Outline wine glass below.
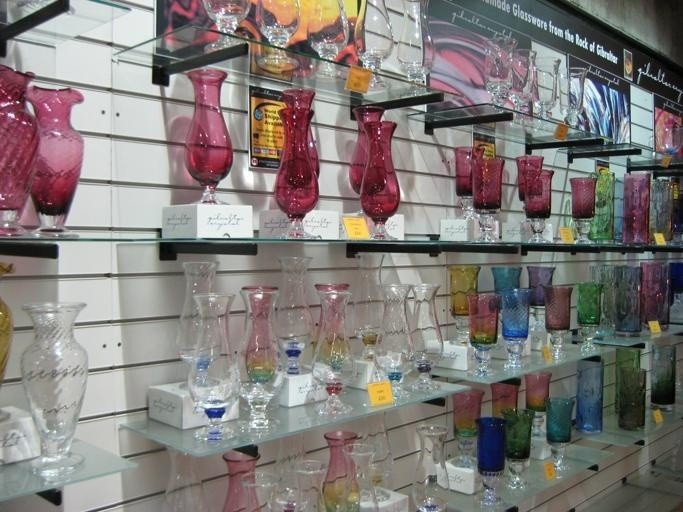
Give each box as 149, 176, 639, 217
186, 72, 234, 208
548, 398, 576, 477
491, 378, 521, 422
239, 286, 282, 437
447, 141, 599, 248
540, 284, 572, 356
350, 106, 385, 217
373, 284, 414, 402
311, 278, 354, 418
469, 294, 498, 385
0, 61, 39, 238
576, 360, 602, 439
273, 107, 326, 243
257, 4, 301, 73
189, 294, 239, 444
405, 285, 439, 395
503, 407, 535, 493
499, 287, 529, 369
576, 283, 602, 365
352, 248, 388, 369
283, 89, 321, 212
306, 1, 351, 81
359, 122, 401, 242
272, 253, 314, 373
355, 3, 393, 91
524, 372, 553, 416
22, 86, 87, 240
201, 1, 253, 53
490, 266, 524, 324
179, 261, 220, 390
19, 301, 91, 477
472, 416, 507, 510
445, 264, 480, 344
0, 1, 603, 512
149, 425, 455, 512
450, 390, 481, 470
397, 2, 438, 98
524, 266, 555, 333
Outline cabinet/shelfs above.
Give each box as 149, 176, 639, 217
0, 1, 683, 512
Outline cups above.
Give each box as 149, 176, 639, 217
592, 175, 675, 435
